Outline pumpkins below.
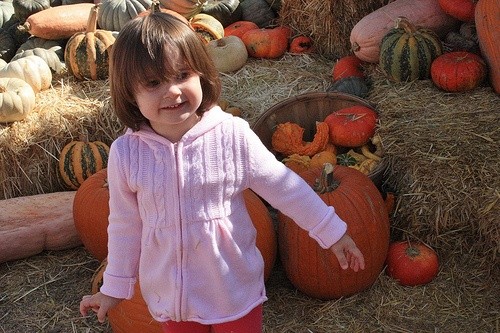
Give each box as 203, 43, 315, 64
0, 105, 438, 333
0, 0, 316, 125
327, 0, 500, 102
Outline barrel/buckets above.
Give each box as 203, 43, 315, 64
251, 92, 389, 190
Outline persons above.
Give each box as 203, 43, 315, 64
79, 13, 366, 333
